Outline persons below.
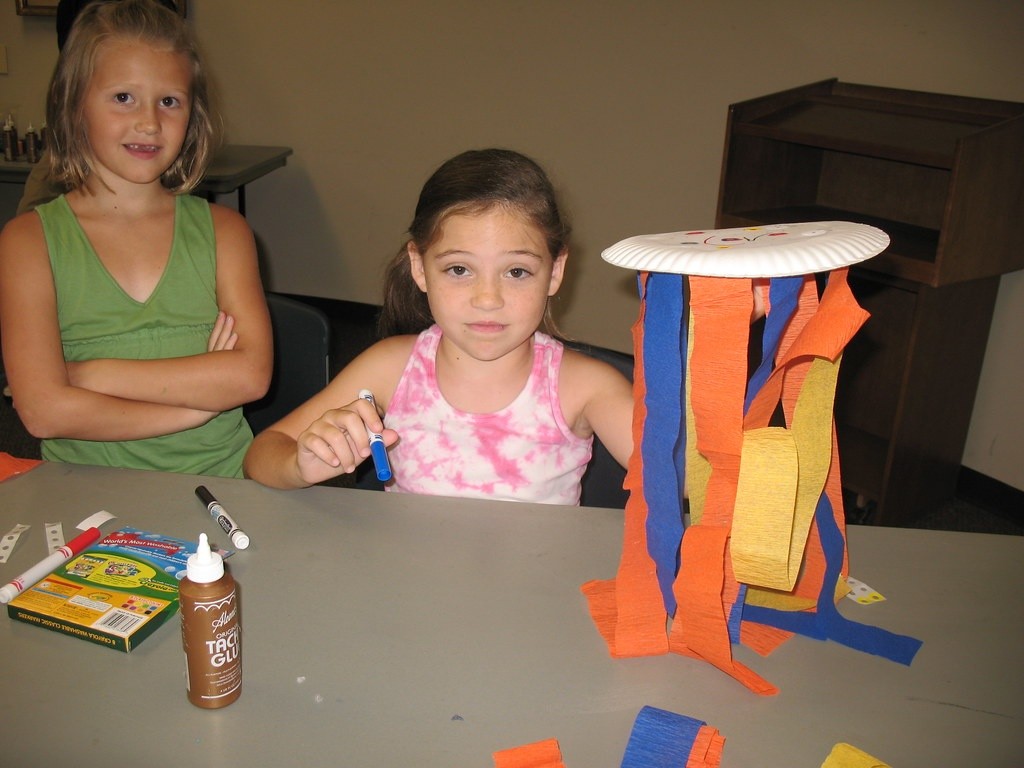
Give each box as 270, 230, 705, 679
17, 0, 189, 220
0, 0, 274, 480
241, 144, 767, 509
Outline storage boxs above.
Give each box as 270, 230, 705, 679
7, 524, 236, 655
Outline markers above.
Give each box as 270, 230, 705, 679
0, 527, 101, 604
194, 485, 250, 550
358, 388, 393, 482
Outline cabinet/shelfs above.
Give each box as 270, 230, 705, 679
714, 77, 1024, 525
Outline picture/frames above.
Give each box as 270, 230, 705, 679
15, 0, 187, 19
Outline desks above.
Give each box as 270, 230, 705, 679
0, 452, 1024, 768
0, 144, 294, 216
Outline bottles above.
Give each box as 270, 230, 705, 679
179, 534, 242, 709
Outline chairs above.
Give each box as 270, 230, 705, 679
16, 293, 343, 489
355, 335, 635, 509
